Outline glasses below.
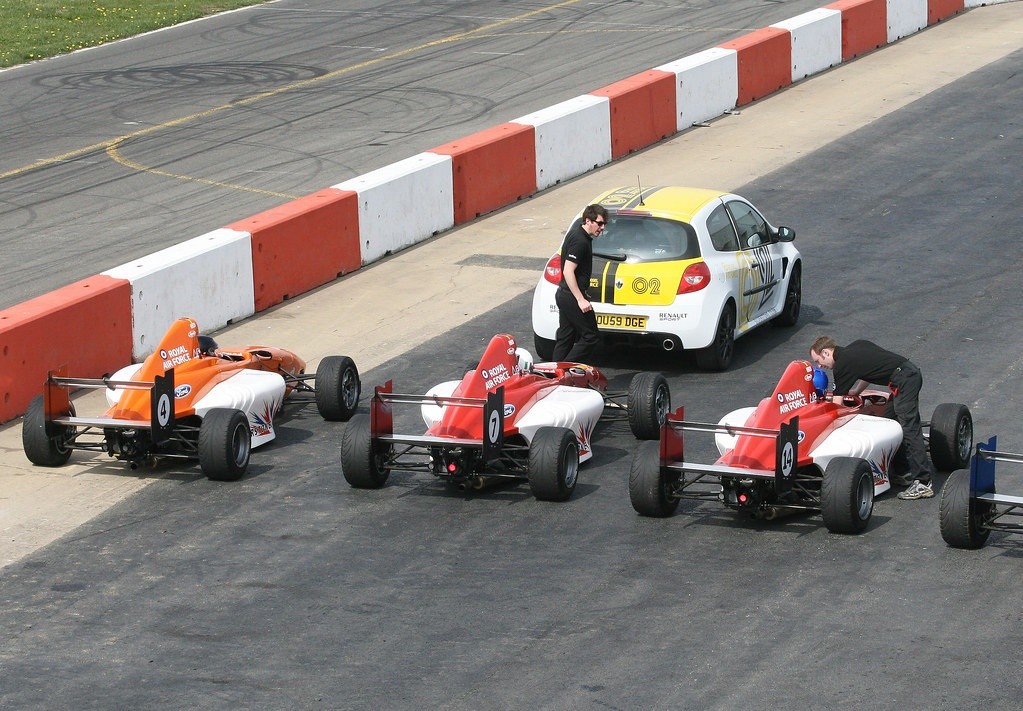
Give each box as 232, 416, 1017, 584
594, 220, 607, 226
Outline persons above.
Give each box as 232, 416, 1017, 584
514, 348, 533, 371
810, 337, 934, 500
198, 336, 218, 357
553, 204, 608, 363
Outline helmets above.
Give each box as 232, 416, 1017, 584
197, 336, 219, 357
515, 347, 534, 372
813, 368, 829, 396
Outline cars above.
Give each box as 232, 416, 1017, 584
531, 186, 802, 372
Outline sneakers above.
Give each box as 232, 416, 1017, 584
896, 479, 934, 499
889, 470, 912, 486
